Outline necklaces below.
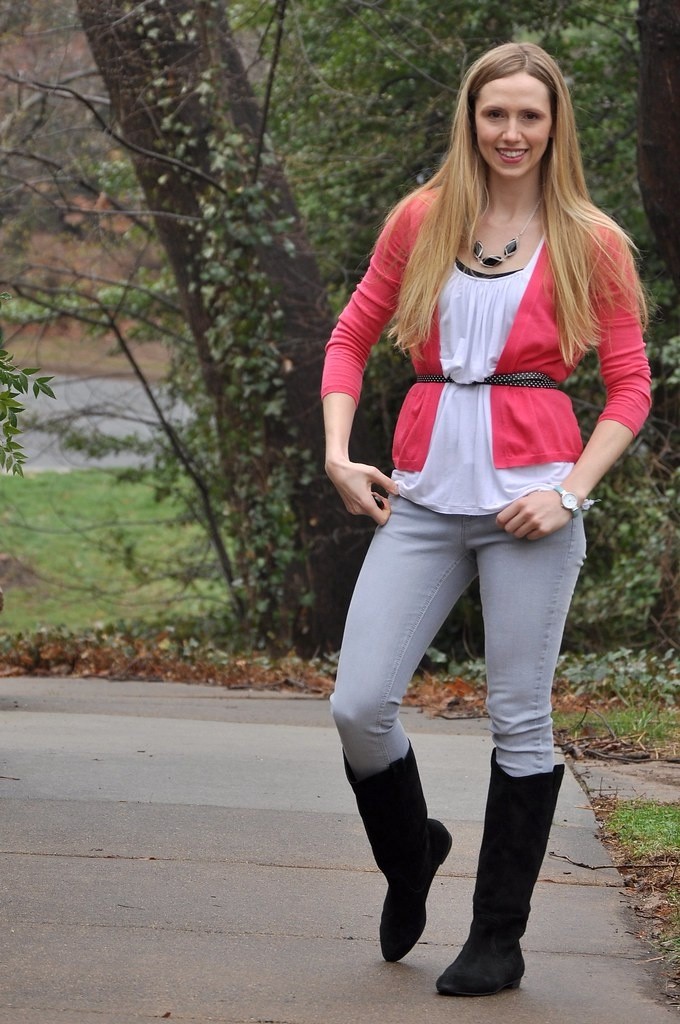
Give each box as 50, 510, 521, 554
472, 195, 540, 268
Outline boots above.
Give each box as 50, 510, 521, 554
436, 747, 565, 996
342, 737, 452, 963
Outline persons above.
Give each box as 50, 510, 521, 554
320, 42, 653, 994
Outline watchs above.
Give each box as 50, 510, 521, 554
553, 485, 581, 518
